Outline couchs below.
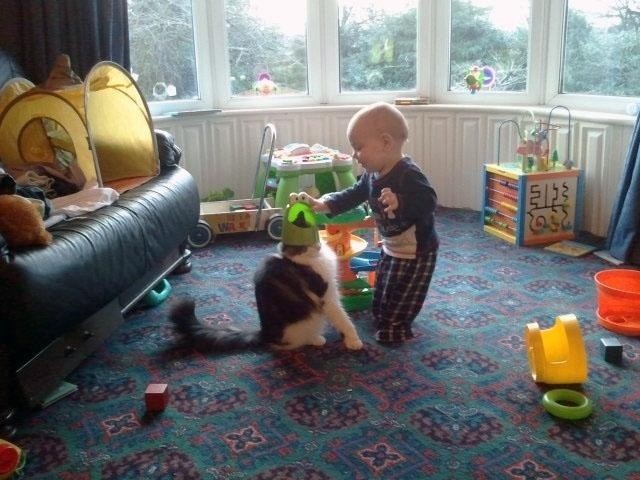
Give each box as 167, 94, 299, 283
1, 47, 201, 415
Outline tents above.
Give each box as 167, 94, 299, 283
0, 61, 162, 191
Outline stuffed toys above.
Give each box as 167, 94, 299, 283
466, 65, 494, 95
0, 195, 52, 251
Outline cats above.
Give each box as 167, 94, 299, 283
164, 211, 364, 356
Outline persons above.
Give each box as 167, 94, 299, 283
309, 102, 440, 344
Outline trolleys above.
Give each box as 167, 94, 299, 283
186, 124, 282, 246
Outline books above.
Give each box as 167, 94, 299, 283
544, 240, 598, 257
